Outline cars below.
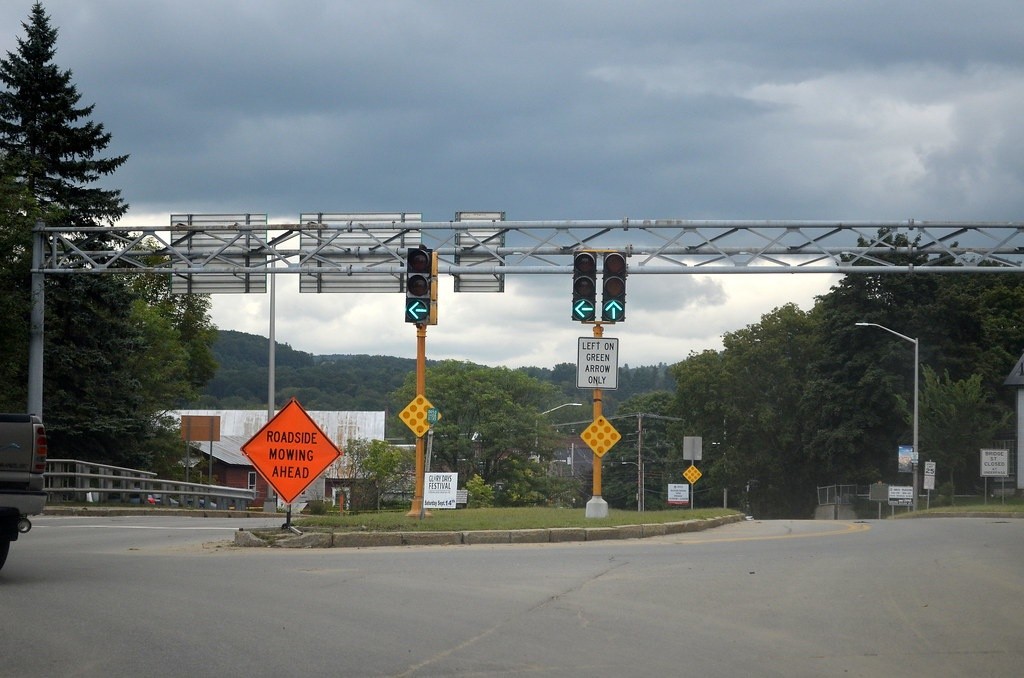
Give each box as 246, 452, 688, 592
58, 491, 217, 510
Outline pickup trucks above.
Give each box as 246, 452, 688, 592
0, 412, 49, 570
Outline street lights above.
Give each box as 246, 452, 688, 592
535, 402, 583, 446
572, 249, 627, 324
855, 322, 920, 513
404, 246, 433, 324
